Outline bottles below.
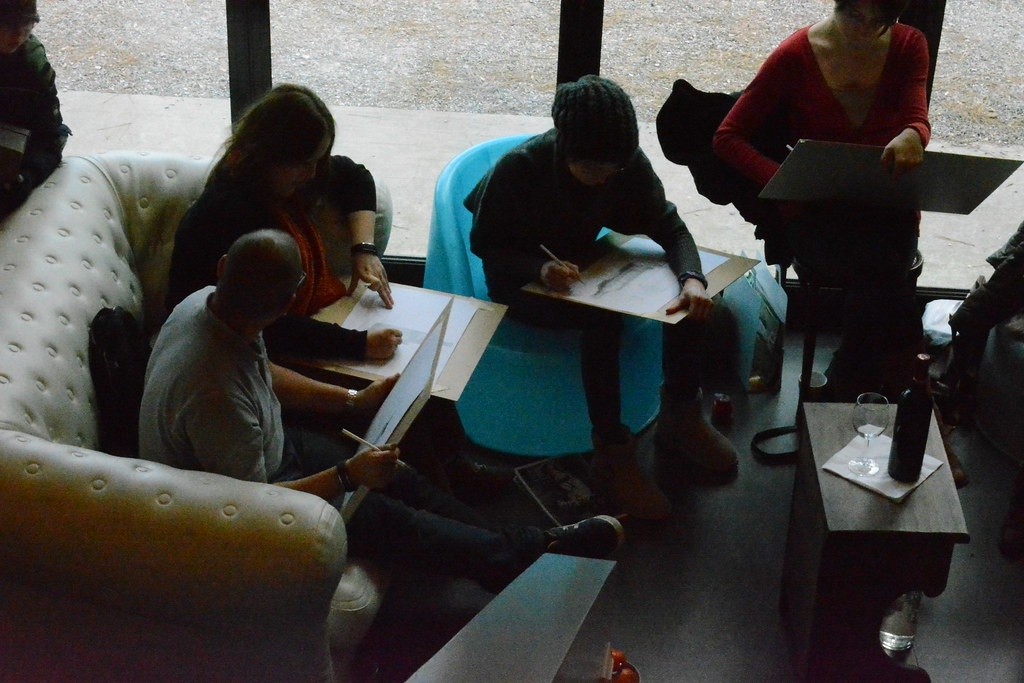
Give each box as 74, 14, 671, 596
888, 352, 934, 483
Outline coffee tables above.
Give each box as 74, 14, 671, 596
784, 396, 971, 681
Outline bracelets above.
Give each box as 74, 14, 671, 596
350, 242, 378, 255
677, 270, 708, 285
337, 460, 355, 494
343, 389, 358, 420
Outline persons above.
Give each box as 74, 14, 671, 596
463, 74, 742, 519
1, 0, 72, 228
138, 230, 626, 599
714, 0, 972, 490
165, 82, 405, 367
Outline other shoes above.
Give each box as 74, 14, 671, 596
654, 382, 740, 474
589, 426, 667, 525
828, 339, 966, 487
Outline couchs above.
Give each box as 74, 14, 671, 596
0, 151, 394, 683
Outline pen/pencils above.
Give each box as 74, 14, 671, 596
341, 428, 412, 471
539, 243, 585, 284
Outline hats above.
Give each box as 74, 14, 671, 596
553, 74, 641, 166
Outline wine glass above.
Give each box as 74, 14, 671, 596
848, 392, 891, 476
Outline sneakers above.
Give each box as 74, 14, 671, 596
546, 514, 625, 561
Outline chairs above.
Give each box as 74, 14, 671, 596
766, 243, 926, 422
420, 130, 664, 458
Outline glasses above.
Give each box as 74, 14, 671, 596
297, 270, 306, 288
